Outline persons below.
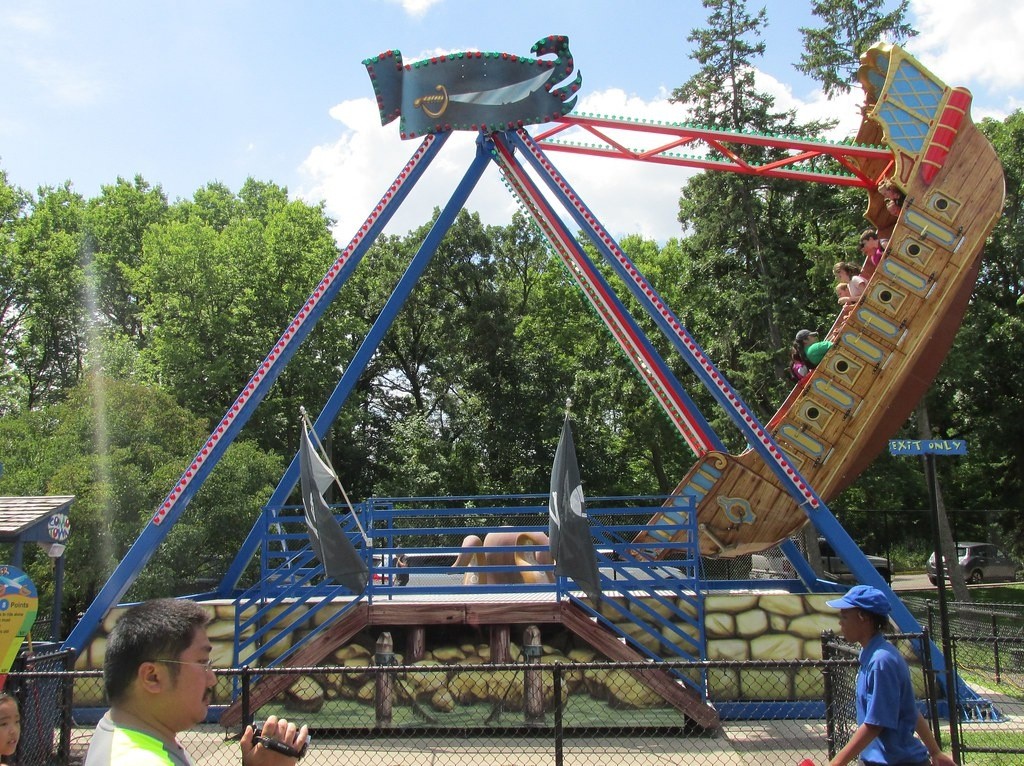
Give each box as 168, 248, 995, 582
785, 176, 906, 379
84, 596, 308, 766
392, 544, 410, 586
0, 693, 22, 766
823, 584, 958, 766
299, 419, 371, 597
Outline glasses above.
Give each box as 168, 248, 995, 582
149, 659, 213, 673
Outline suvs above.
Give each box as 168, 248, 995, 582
752, 536, 893, 586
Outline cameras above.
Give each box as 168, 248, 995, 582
251, 721, 312, 759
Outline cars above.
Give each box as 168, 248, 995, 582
926, 542, 1024, 586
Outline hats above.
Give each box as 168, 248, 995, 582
825, 584, 891, 616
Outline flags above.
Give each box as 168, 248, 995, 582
548, 413, 602, 606
